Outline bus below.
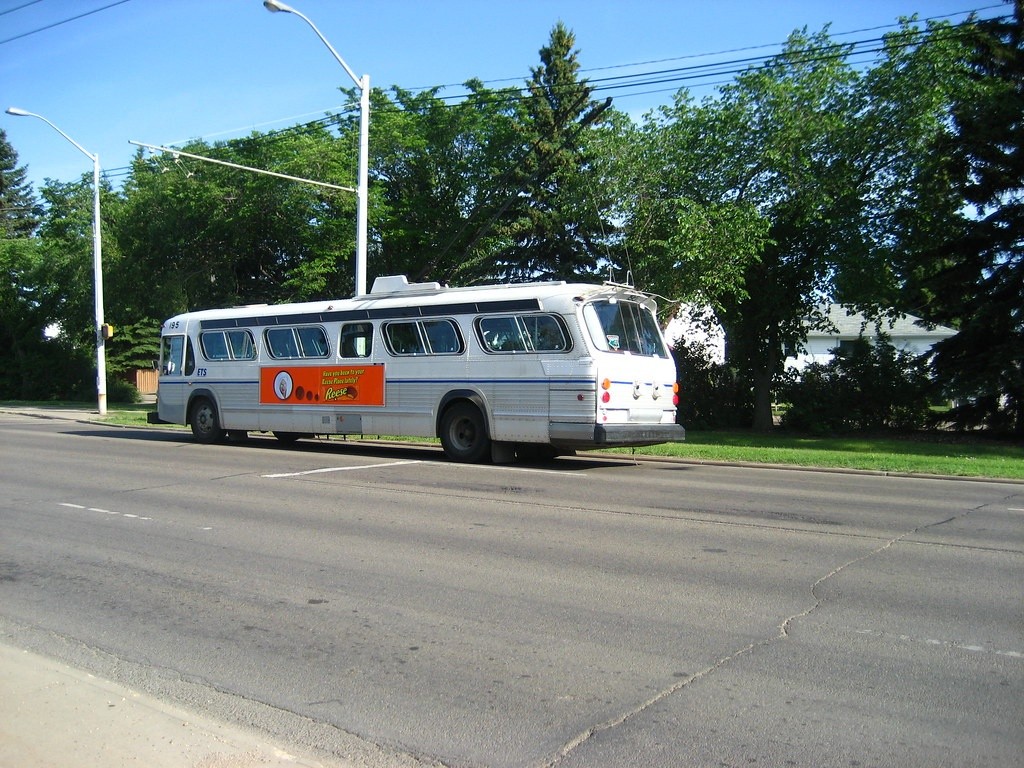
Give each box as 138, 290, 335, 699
145, 273, 687, 465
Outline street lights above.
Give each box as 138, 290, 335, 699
261, 0, 371, 358
5, 107, 108, 418
